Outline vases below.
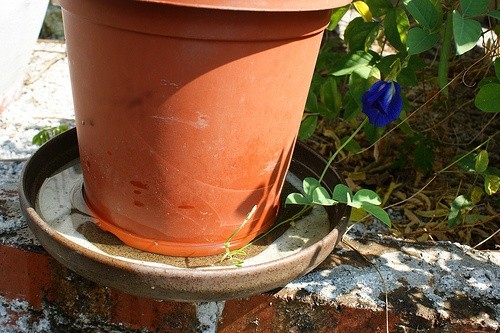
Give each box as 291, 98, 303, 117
58, 0, 354, 257
